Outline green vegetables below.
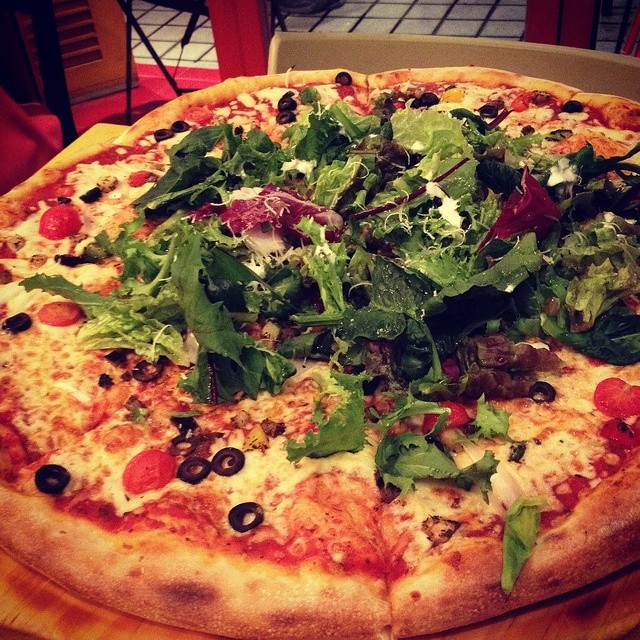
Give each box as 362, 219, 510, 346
17, 86, 640, 596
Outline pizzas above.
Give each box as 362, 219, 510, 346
0, 65, 640, 639
